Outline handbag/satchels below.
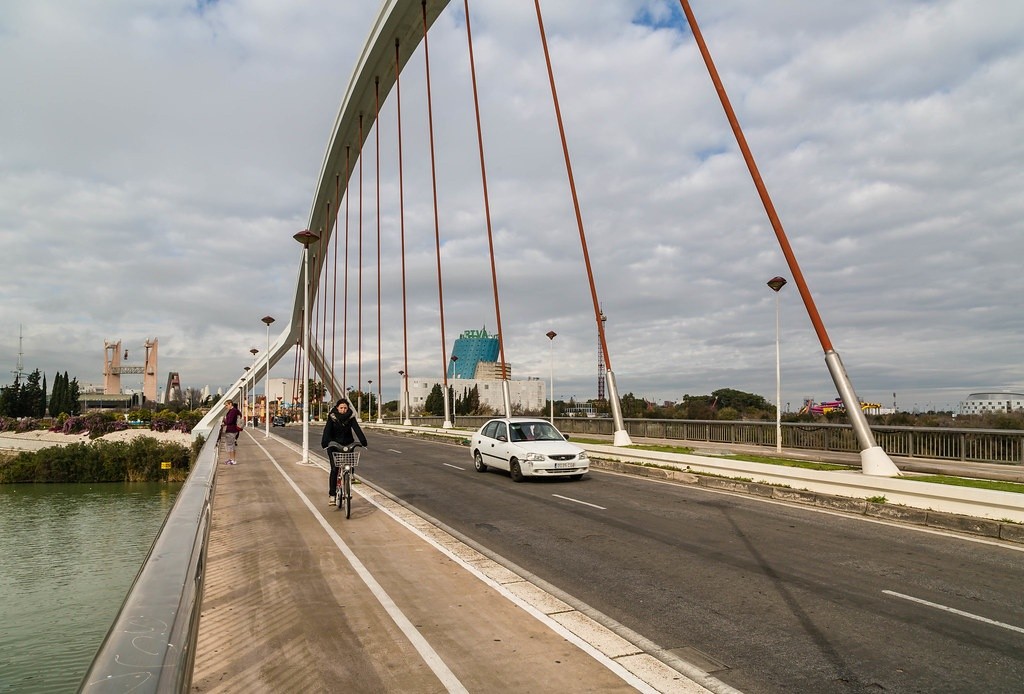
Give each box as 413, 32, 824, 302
236, 414, 244, 432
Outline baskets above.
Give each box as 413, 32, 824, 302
332, 452, 360, 467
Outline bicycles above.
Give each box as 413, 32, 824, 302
323, 442, 368, 519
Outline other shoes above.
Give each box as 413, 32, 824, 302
328, 495, 336, 506
351, 478, 361, 484
224, 460, 236, 465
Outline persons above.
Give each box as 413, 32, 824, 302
321, 399, 366, 506
222, 399, 242, 464
530, 424, 543, 439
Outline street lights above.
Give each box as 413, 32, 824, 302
244, 367, 251, 426
368, 379, 373, 422
398, 370, 404, 424
250, 348, 259, 427
546, 330, 557, 426
260, 315, 275, 438
239, 386, 242, 412
451, 355, 458, 415
766, 276, 788, 453
241, 378, 246, 422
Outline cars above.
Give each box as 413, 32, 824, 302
272, 417, 285, 427
470, 418, 590, 484
